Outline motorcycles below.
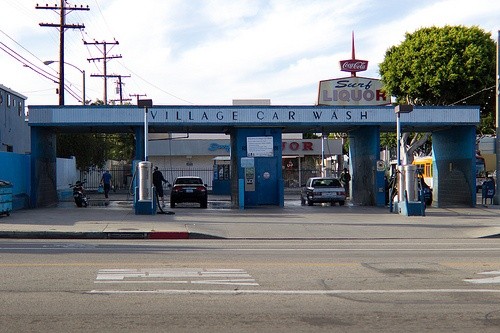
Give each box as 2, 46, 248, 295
69, 178, 88, 208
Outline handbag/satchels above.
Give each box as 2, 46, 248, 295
98, 184, 104, 193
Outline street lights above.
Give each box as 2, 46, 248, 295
43, 60, 86, 105
395, 103, 414, 165
137, 99, 153, 160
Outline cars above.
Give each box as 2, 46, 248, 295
168, 176, 208, 209
417, 177, 433, 206
301, 176, 346, 206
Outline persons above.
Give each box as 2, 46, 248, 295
384, 176, 390, 206
340, 168, 350, 197
100, 170, 114, 198
153, 167, 168, 207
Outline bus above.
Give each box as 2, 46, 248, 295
390, 150, 487, 193
